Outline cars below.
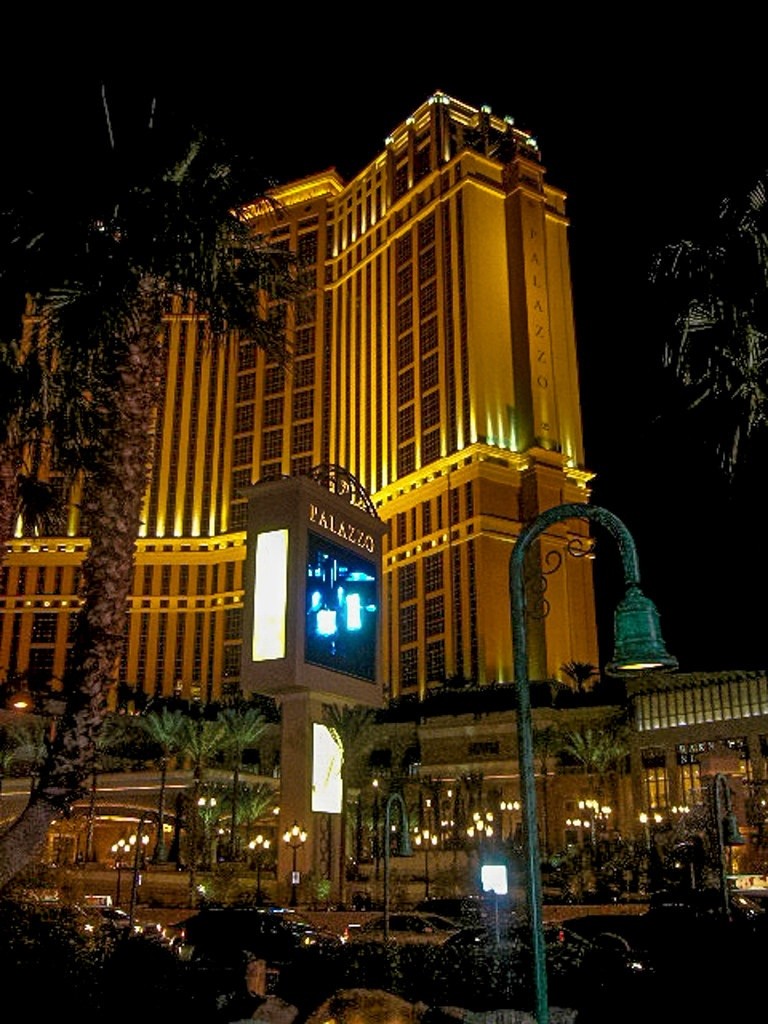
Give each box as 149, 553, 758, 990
166, 907, 359, 987
429, 919, 658, 1007
1, 889, 229, 1023
343, 909, 459, 943
411, 897, 489, 925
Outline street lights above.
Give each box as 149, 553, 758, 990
468, 809, 496, 896
247, 835, 273, 908
508, 500, 681, 1024
381, 793, 417, 942
110, 838, 131, 906
710, 771, 747, 930
283, 820, 311, 908
414, 824, 439, 901
128, 806, 170, 935
